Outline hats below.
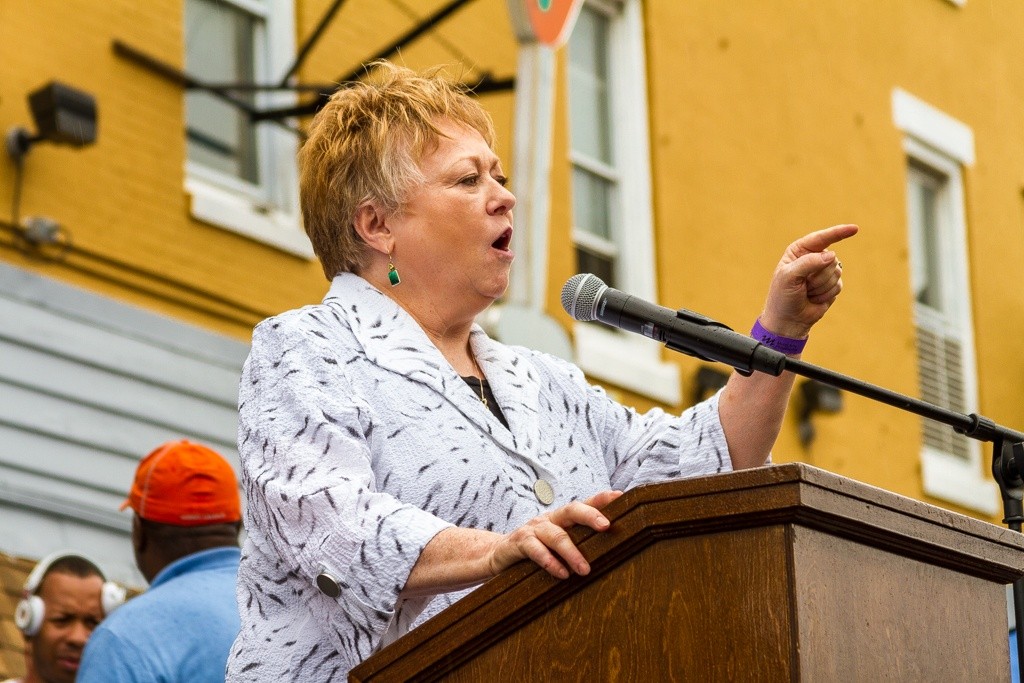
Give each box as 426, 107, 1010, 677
120, 439, 242, 525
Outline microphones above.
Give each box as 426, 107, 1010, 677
560, 273, 787, 378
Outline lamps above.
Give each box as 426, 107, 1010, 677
797, 380, 842, 446
7, 83, 96, 158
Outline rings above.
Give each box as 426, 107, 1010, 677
838, 260, 844, 269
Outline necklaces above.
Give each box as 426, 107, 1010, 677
453, 352, 488, 408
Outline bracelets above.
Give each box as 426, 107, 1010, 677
750, 317, 809, 356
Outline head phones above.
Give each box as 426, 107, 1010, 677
14, 552, 126, 637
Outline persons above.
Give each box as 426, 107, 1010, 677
0, 555, 127, 683
221, 60, 858, 683
76, 441, 249, 683
